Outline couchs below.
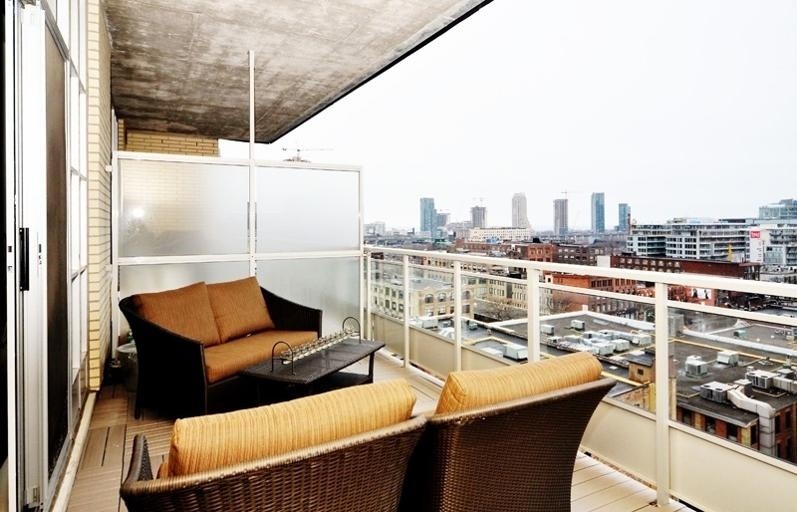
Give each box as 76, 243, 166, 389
118, 277, 322, 420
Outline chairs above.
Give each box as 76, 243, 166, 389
399, 377, 620, 512
120, 414, 428, 512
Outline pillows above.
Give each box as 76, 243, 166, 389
435, 353, 602, 419
169, 380, 417, 472
132, 276, 273, 349
